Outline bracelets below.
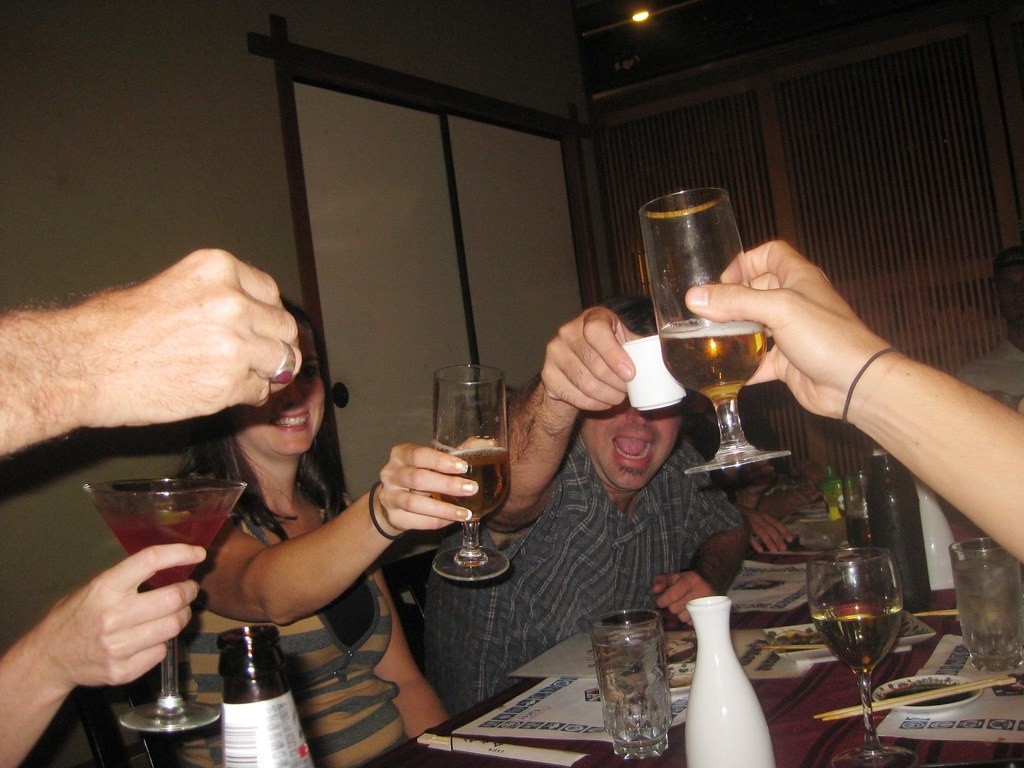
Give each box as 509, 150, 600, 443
842, 348, 899, 424
368, 481, 404, 539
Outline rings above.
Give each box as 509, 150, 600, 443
268, 338, 296, 384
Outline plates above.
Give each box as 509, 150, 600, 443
872, 675, 982, 719
763, 611, 936, 661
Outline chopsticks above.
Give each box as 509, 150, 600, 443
755, 644, 831, 649
813, 676, 1016, 722
912, 609, 958, 616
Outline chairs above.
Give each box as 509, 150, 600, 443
69, 548, 439, 768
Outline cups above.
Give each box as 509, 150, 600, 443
622, 334, 687, 411
949, 537, 1024, 675
590, 608, 673, 760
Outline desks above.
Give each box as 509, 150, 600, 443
349, 512, 1024, 768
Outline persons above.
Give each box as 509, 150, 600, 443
174, 294, 479, 767
686, 237, 1023, 566
415, 295, 817, 715
1, 247, 306, 453
957, 245, 1024, 398
0, 543, 206, 768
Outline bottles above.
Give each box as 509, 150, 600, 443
913, 476, 958, 590
841, 469, 876, 551
818, 466, 847, 521
216, 624, 318, 768
864, 453, 931, 614
685, 595, 777, 767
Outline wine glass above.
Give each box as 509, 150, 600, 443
638, 187, 791, 475
82, 478, 249, 732
431, 364, 511, 582
808, 547, 918, 768
